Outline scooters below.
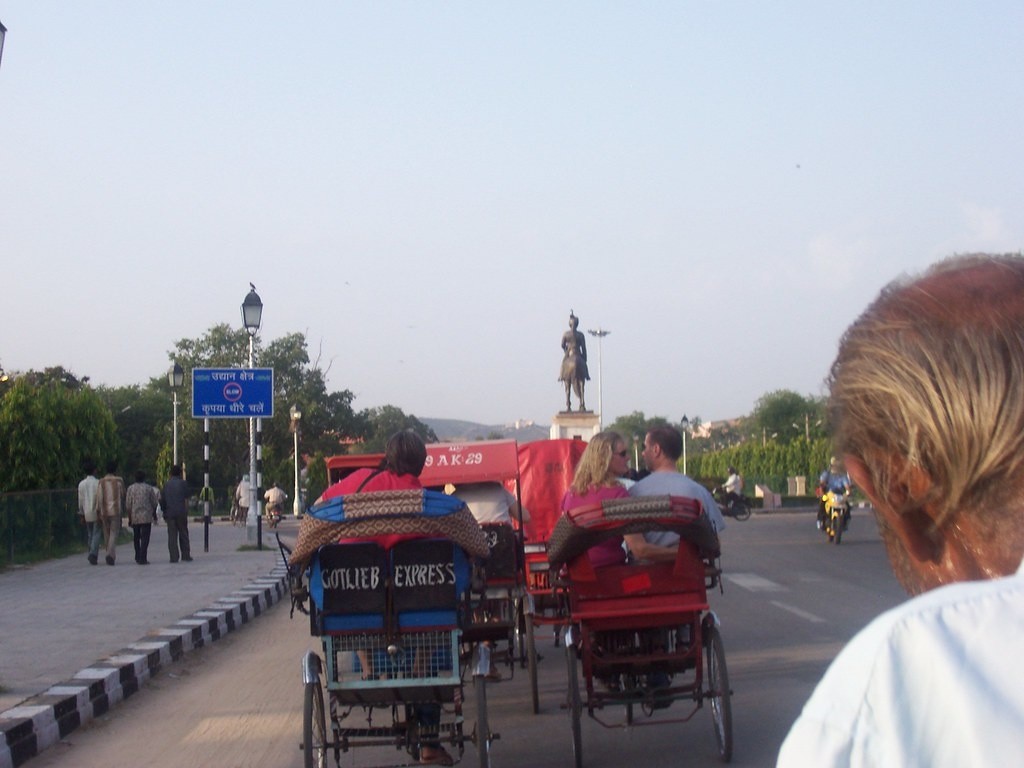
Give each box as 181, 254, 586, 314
268, 492, 288, 529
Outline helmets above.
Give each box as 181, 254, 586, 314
274, 482, 279, 487
830, 456, 837, 467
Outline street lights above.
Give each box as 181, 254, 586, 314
792, 413, 823, 441
681, 414, 690, 475
166, 362, 185, 466
632, 431, 641, 473
240, 282, 264, 550
587, 328, 611, 434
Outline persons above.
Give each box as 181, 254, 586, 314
150, 479, 162, 515
816, 456, 851, 528
125, 470, 158, 565
718, 466, 743, 508
628, 426, 725, 685
560, 432, 676, 567
451, 482, 530, 531
236, 475, 250, 508
162, 465, 193, 563
264, 482, 288, 519
78, 464, 100, 564
776, 252, 1024, 768
311, 432, 456, 768
95, 462, 126, 566
558, 315, 590, 412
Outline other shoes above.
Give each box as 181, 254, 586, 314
418, 745, 454, 765
817, 520, 824, 530
361, 674, 377, 681
648, 671, 672, 710
182, 554, 193, 561
105, 555, 114, 565
136, 558, 147, 564
88, 555, 97, 565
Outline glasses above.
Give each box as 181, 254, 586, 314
614, 449, 626, 458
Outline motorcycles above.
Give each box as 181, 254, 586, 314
817, 487, 854, 543
710, 486, 751, 521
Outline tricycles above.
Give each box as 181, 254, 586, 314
292, 439, 737, 768
